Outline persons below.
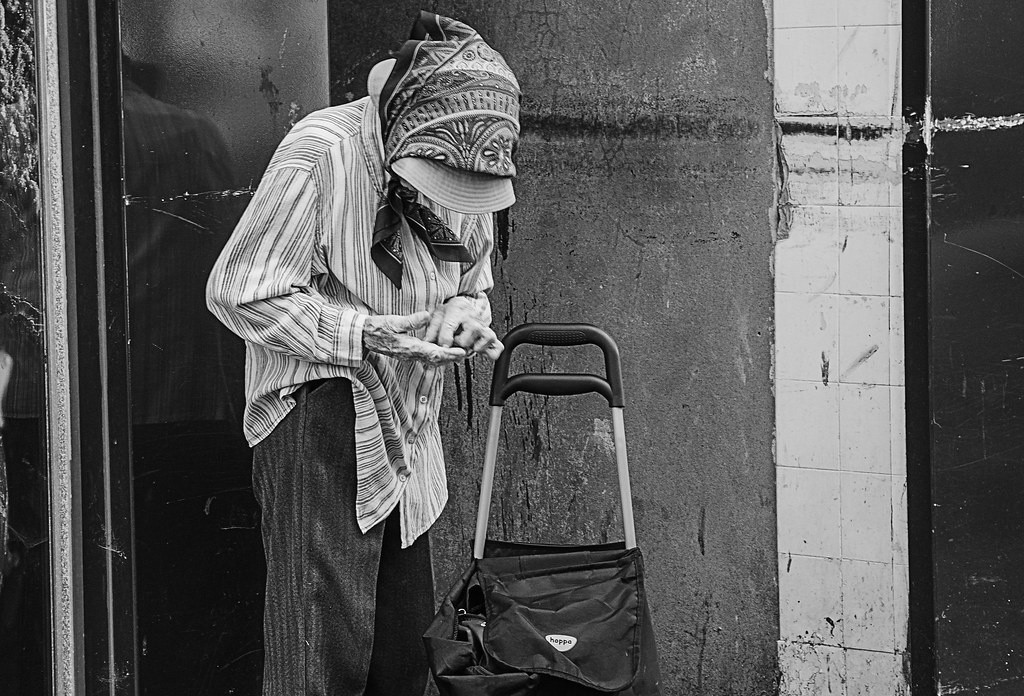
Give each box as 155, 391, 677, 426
204, 9, 525, 695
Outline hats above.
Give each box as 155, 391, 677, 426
368, 59, 517, 213
